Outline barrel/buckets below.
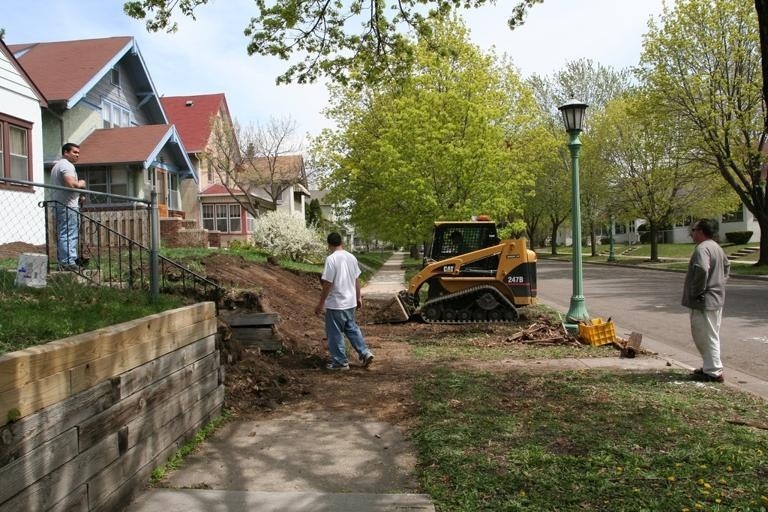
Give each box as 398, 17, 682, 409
14, 253, 49, 290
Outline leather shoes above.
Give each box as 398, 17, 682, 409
693, 368, 724, 383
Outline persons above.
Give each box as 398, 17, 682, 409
315, 233, 374, 370
680, 218, 731, 384
441, 230, 471, 268
48, 143, 88, 271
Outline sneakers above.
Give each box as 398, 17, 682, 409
56, 264, 84, 271
361, 352, 374, 368
326, 362, 350, 371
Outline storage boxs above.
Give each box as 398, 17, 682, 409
579, 318, 617, 348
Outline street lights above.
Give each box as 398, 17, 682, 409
606, 199, 617, 261
557, 90, 591, 335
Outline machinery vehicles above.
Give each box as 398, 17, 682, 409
367, 220, 537, 325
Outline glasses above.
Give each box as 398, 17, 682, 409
691, 228, 702, 231
